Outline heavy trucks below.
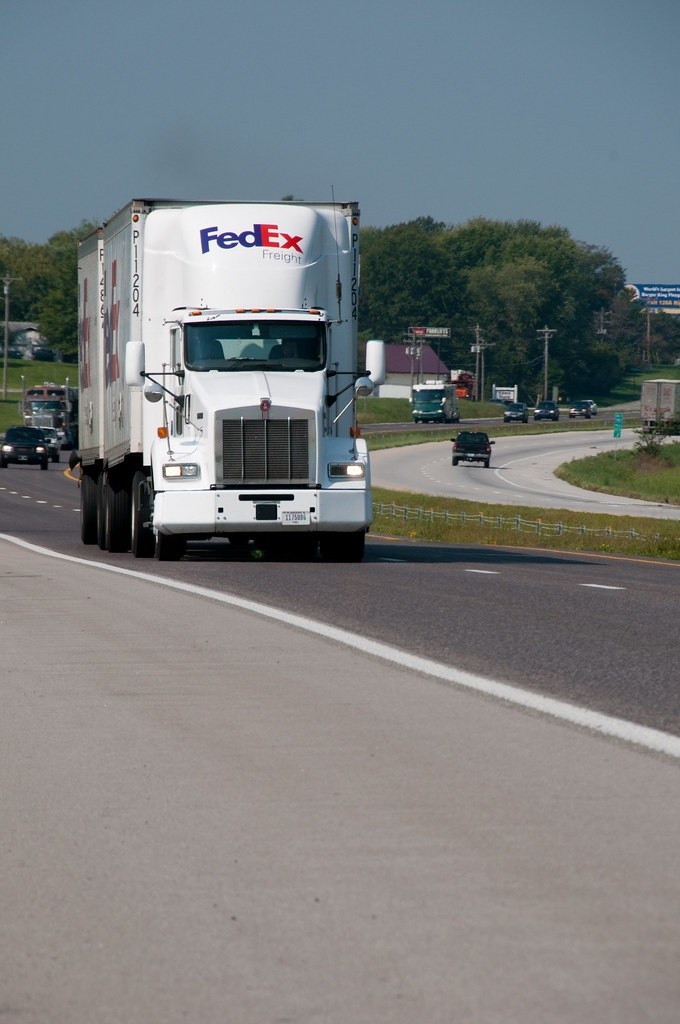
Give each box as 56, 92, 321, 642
73, 197, 390, 561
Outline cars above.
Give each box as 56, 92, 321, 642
449, 430, 496, 470
569, 400, 592, 420
582, 400, 599, 415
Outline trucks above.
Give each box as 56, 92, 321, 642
411, 379, 461, 424
19, 382, 78, 451
640, 379, 680, 434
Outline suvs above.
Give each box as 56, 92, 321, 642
534, 400, 562, 422
0, 427, 51, 471
504, 402, 530, 423
26, 425, 62, 463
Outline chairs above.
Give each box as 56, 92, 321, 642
268, 345, 301, 359
193, 341, 224, 360
